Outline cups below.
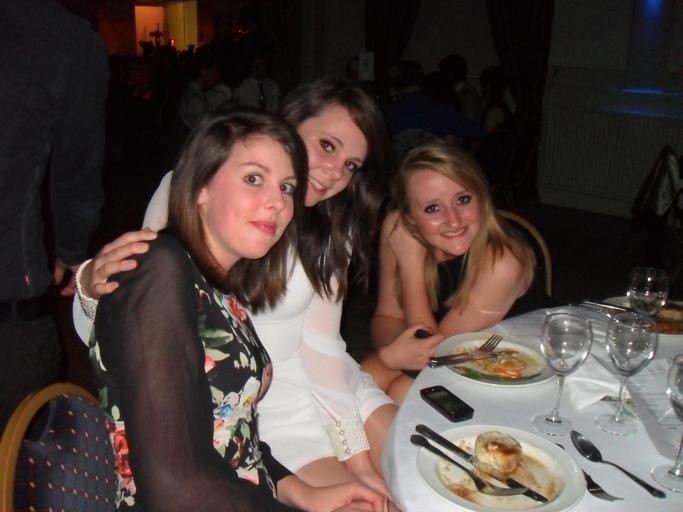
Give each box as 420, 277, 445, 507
628, 266, 669, 318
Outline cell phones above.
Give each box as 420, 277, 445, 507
420, 384, 475, 422
400, 329, 433, 378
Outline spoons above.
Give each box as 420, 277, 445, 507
570, 429, 666, 499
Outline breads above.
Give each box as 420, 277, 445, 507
475, 431, 522, 474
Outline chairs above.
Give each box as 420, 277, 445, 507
480, 110, 541, 206
1, 382, 122, 512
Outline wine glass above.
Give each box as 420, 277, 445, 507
597, 311, 660, 436
652, 351, 683, 493
532, 312, 594, 437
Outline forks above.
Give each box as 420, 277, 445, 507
429, 333, 503, 363
403, 432, 528, 498
553, 441, 625, 502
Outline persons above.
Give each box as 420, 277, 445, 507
345, 45, 518, 206
366, 142, 536, 407
2, 3, 112, 509
71, 78, 397, 512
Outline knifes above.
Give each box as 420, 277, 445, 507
415, 423, 549, 504
426, 349, 520, 369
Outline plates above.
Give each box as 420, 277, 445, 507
600, 295, 683, 338
436, 331, 558, 387
401, 424, 587, 512
